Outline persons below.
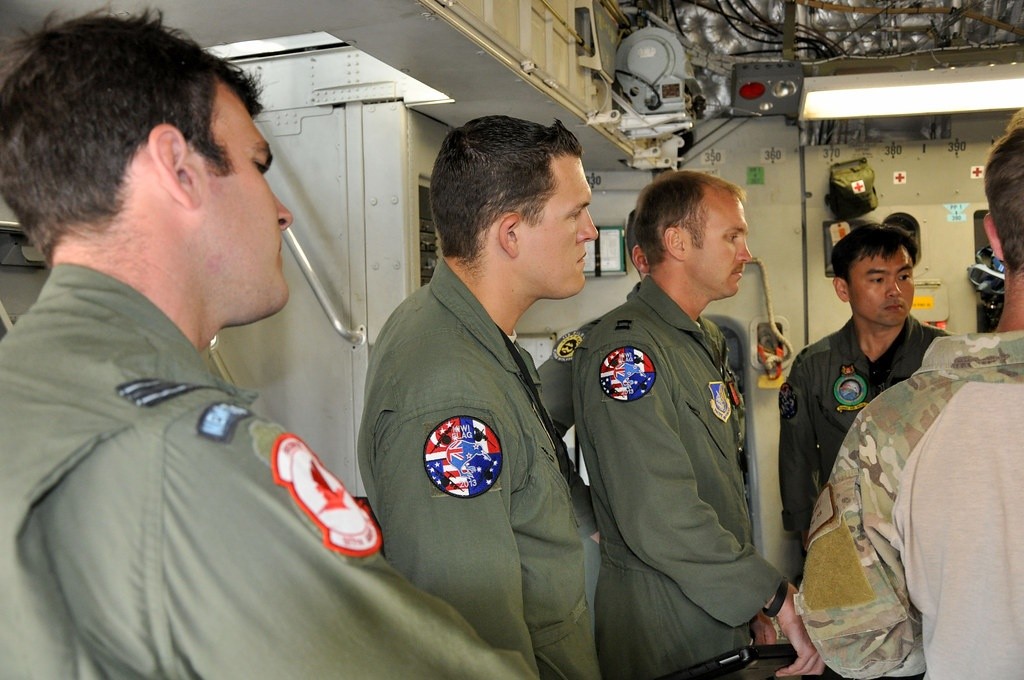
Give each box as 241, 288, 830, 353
537, 210, 651, 542
1, 13, 540, 680
358, 116, 601, 680
794, 105, 1024, 680
572, 171, 823, 680
778, 224, 953, 551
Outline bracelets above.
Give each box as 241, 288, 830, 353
762, 575, 788, 617
